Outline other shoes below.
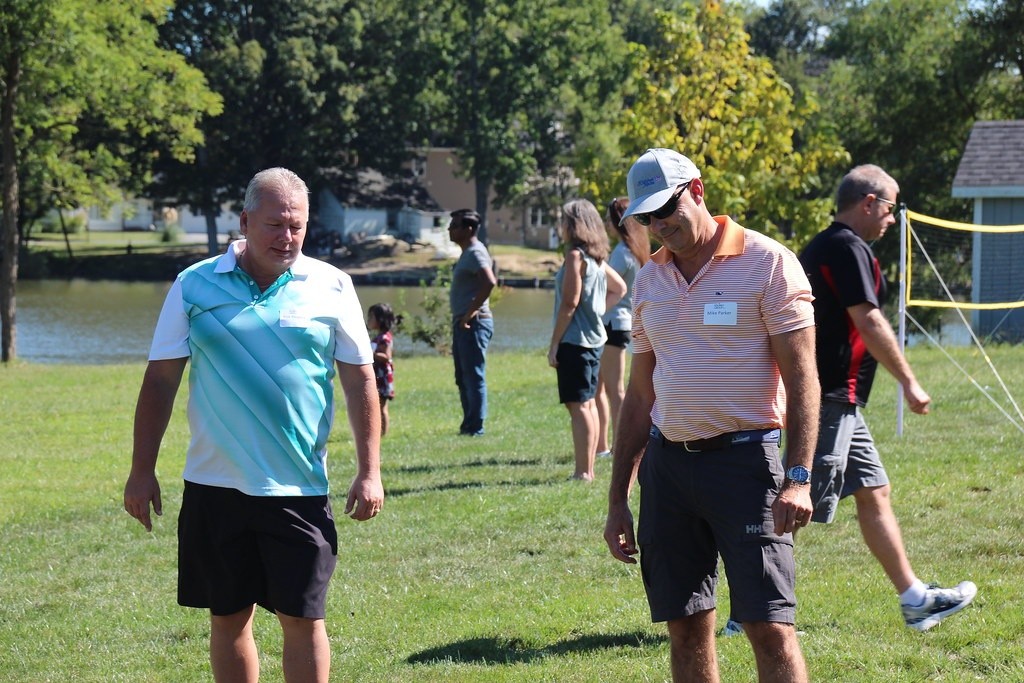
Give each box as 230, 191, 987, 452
596, 449, 611, 458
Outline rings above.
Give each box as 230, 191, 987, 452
796, 520, 802, 523
374, 509, 381, 512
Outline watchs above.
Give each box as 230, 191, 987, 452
786, 465, 811, 485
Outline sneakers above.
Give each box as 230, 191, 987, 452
903, 580, 978, 631
724, 621, 741, 636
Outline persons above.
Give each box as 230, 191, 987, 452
124, 166, 384, 683
604, 148, 822, 683
721, 165, 978, 636
366, 303, 402, 435
547, 199, 650, 481
447, 209, 497, 435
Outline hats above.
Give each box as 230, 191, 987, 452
619, 148, 702, 226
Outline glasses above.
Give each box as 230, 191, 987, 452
865, 192, 901, 217
633, 183, 690, 226
